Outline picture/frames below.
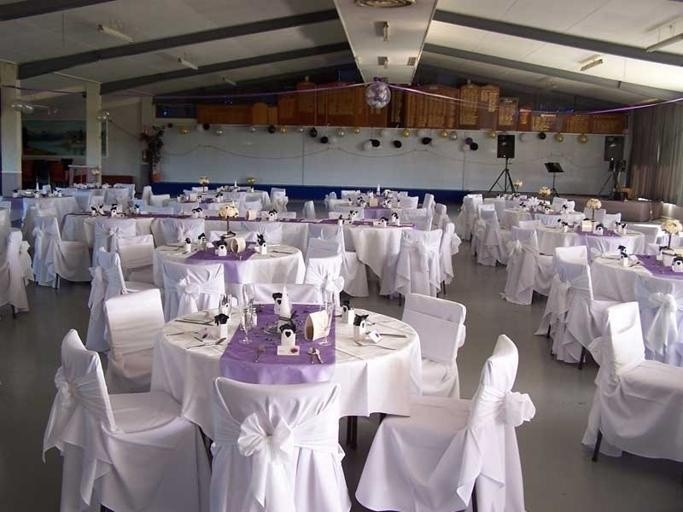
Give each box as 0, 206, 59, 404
22, 117, 109, 161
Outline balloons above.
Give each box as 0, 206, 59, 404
168, 123, 223, 136
488, 130, 564, 144
96, 109, 111, 122
248, 124, 305, 135
578, 134, 588, 144
416, 128, 479, 151
368, 128, 409, 149
309, 127, 360, 144
9, 98, 24, 111
245, 176, 256, 185
22, 101, 34, 114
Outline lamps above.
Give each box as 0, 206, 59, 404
96, 25, 134, 44
177, 57, 198, 72
646, 33, 683, 53
383, 22, 389, 41
580, 59, 602, 72
354, 0, 416, 9
221, 77, 236, 87
384, 58, 389, 69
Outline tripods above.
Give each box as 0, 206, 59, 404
489, 158, 516, 193
598, 161, 622, 200
549, 173, 560, 204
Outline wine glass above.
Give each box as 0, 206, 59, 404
240, 307, 252, 344
241, 282, 256, 330
318, 300, 333, 347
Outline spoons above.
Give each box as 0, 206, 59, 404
174, 317, 215, 325
186, 337, 227, 350
306, 346, 317, 365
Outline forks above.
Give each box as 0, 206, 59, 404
351, 339, 396, 351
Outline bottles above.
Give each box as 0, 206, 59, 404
243, 309, 249, 330
253, 308, 258, 326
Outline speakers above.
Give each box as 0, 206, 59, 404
497, 135, 515, 158
605, 136, 624, 161
